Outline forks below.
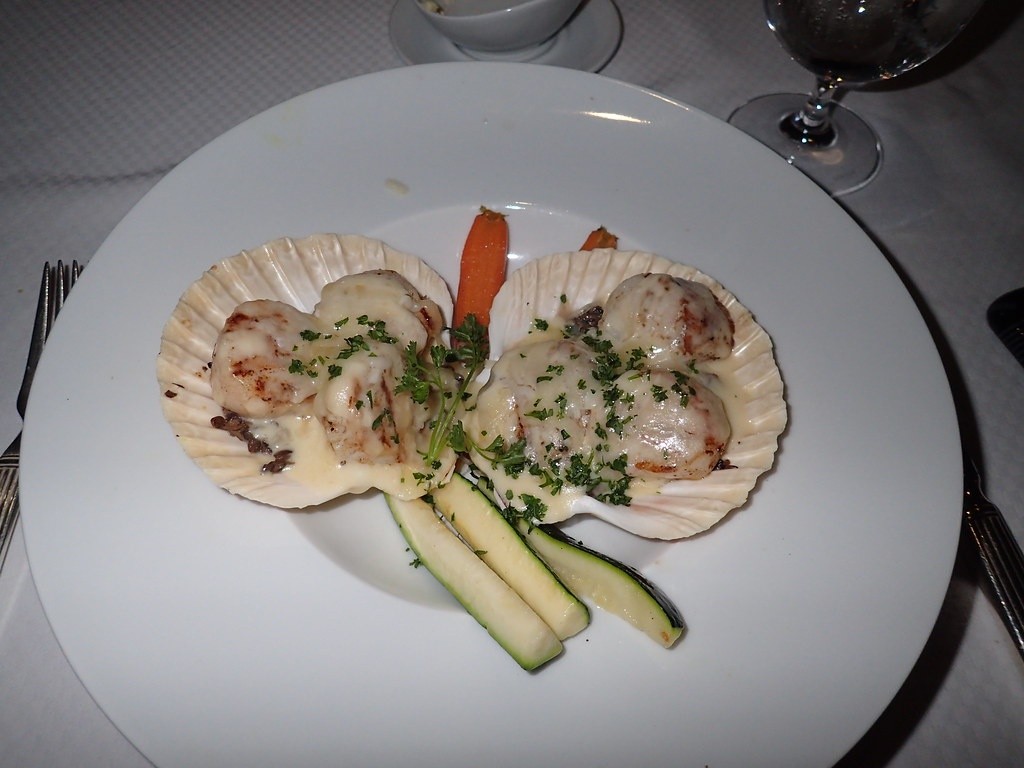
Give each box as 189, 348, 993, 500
0, 259, 79, 565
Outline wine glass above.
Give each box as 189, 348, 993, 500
726, 0, 976, 200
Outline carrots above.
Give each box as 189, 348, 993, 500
452, 212, 508, 355
576, 225, 619, 250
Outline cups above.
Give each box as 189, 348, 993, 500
418, 1, 582, 49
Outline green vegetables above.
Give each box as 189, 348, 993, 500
288, 313, 700, 570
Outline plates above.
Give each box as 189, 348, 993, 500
390, 0, 623, 75
19, 63, 963, 768
